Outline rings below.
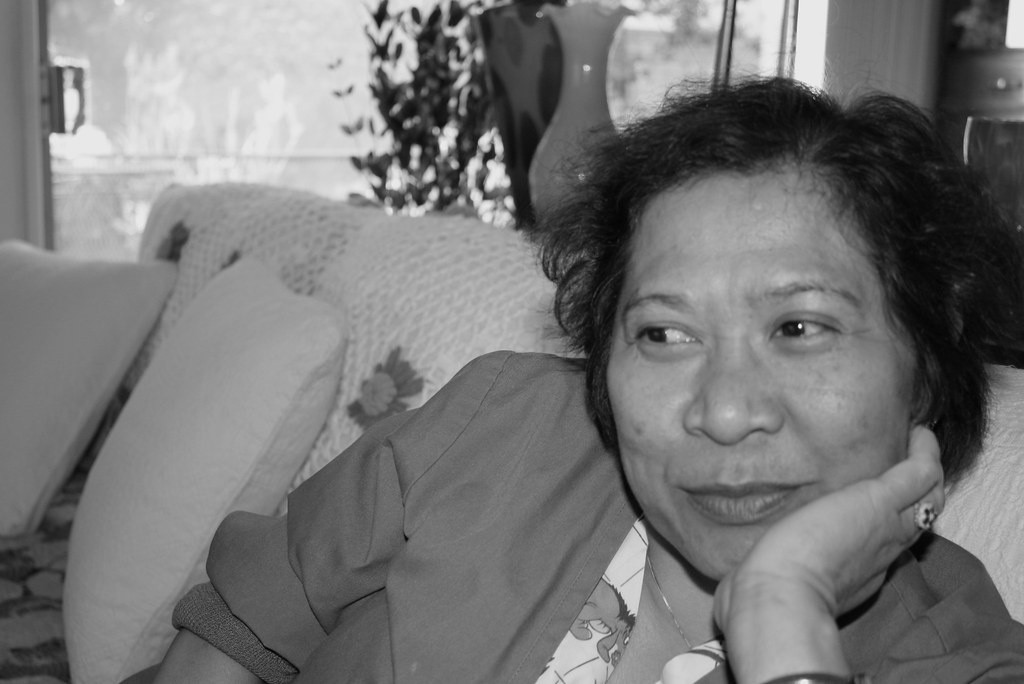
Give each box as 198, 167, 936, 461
913, 502, 938, 531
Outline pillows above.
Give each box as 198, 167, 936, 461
63, 263, 350, 684
0, 238, 180, 536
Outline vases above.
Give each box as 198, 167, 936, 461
478, 0, 567, 221
528, 0, 636, 223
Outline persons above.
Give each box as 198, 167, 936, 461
150, 73, 1024, 684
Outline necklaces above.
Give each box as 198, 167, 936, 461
647, 555, 692, 651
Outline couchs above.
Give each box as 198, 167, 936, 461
0, 184, 1024, 684
